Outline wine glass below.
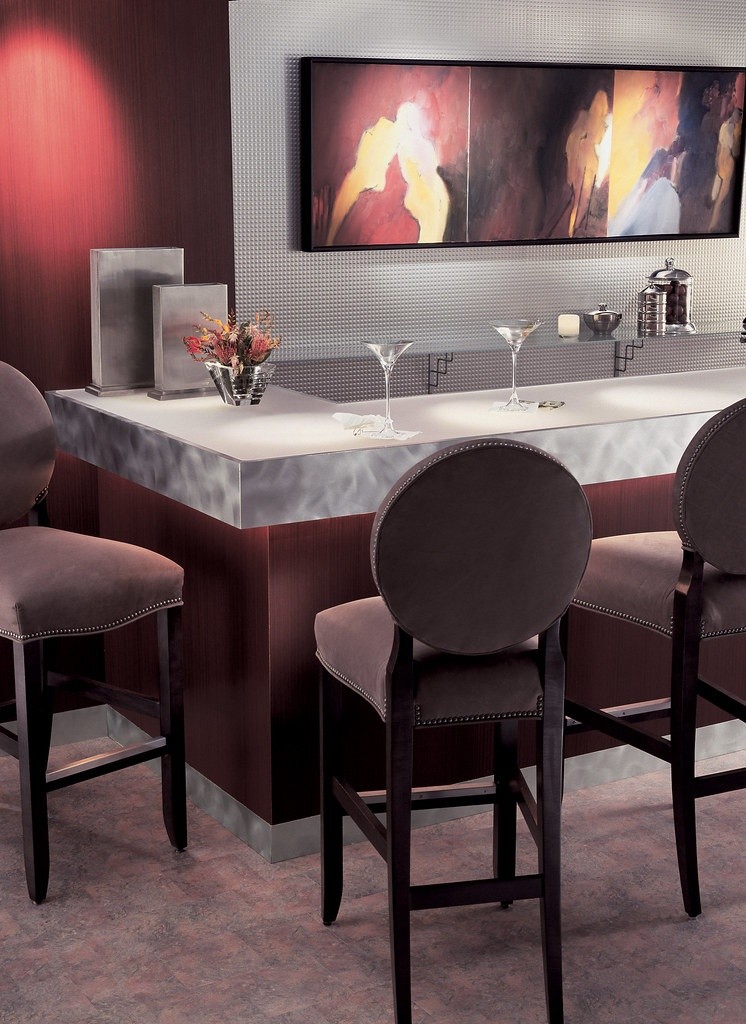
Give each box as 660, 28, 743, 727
360, 339, 415, 435
489, 318, 543, 412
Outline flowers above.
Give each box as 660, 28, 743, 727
182, 310, 283, 366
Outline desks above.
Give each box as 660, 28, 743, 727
43, 366, 746, 864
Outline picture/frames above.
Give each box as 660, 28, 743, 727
300, 56, 746, 254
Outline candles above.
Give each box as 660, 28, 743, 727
558, 313, 580, 336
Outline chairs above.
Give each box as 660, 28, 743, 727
561, 397, 746, 917
312, 437, 595, 1023
0, 359, 187, 905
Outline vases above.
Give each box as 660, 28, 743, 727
203, 360, 278, 406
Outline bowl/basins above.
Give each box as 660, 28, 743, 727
583, 304, 622, 335
